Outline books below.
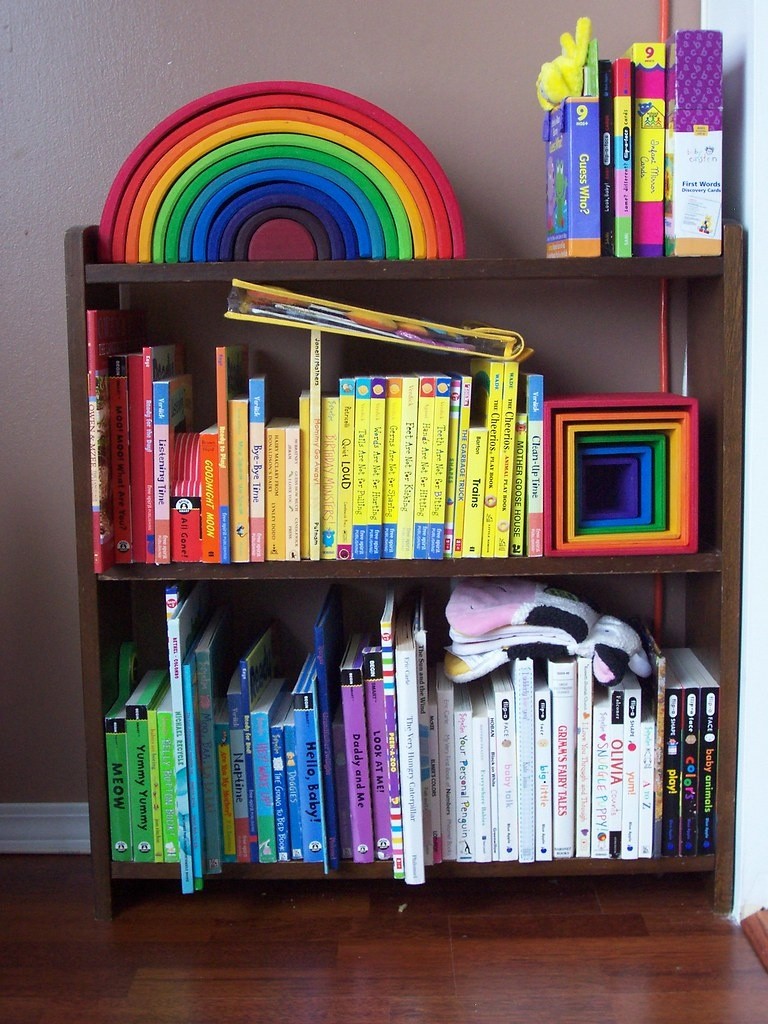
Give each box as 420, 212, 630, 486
85, 277, 544, 574
544, 30, 723, 259
107, 569, 721, 894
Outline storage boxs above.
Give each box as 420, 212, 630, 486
666, 29, 723, 113
662, 109, 723, 257
545, 40, 601, 257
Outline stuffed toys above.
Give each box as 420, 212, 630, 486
443, 576, 651, 687
535, 17, 593, 109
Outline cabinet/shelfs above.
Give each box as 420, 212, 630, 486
64, 218, 743, 922
